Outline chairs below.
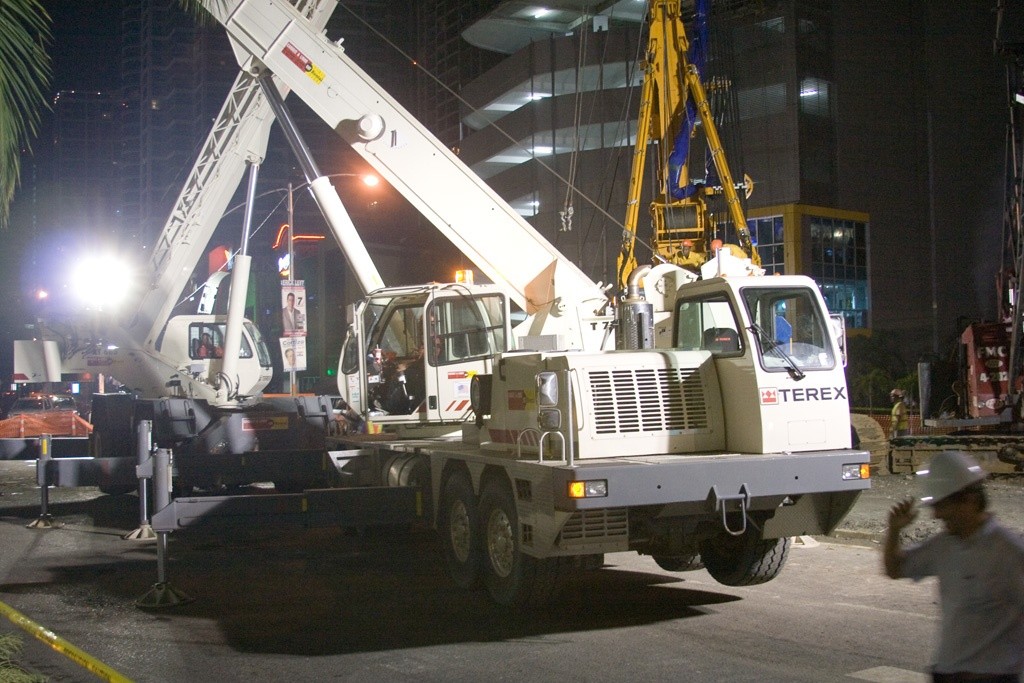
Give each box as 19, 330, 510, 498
701, 326, 741, 355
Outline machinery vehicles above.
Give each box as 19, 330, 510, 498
121, 0, 873, 614
0, 0, 364, 532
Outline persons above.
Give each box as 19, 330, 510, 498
672, 239, 747, 266
282, 293, 302, 330
284, 349, 305, 368
397, 313, 443, 415
885, 450, 1024, 683
198, 333, 216, 358
889, 388, 909, 438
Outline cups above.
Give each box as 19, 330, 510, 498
374, 349, 383, 360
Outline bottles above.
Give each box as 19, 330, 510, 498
97, 373, 105, 394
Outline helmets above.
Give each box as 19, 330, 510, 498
917, 452, 988, 504
891, 389, 902, 397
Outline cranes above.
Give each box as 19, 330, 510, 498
613, 1, 767, 303
